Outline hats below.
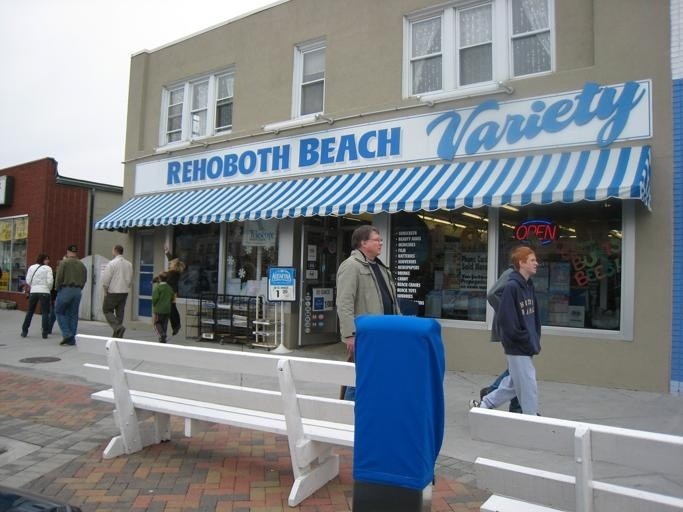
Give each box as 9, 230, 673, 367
66, 245, 79, 252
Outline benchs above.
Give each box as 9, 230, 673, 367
466, 407, 683, 512
75, 334, 355, 507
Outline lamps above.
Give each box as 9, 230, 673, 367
416, 82, 514, 107
152, 139, 209, 155
260, 114, 333, 134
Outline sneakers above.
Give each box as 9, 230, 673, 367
468, 400, 480, 410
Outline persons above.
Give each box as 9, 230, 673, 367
101, 244, 134, 339
47, 260, 64, 335
53, 243, 88, 346
480, 261, 523, 412
335, 224, 403, 401
150, 244, 186, 336
469, 245, 543, 416
151, 270, 175, 344
19, 254, 54, 339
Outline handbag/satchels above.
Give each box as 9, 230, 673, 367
23, 283, 30, 299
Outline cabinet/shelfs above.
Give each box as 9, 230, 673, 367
186, 293, 280, 350
